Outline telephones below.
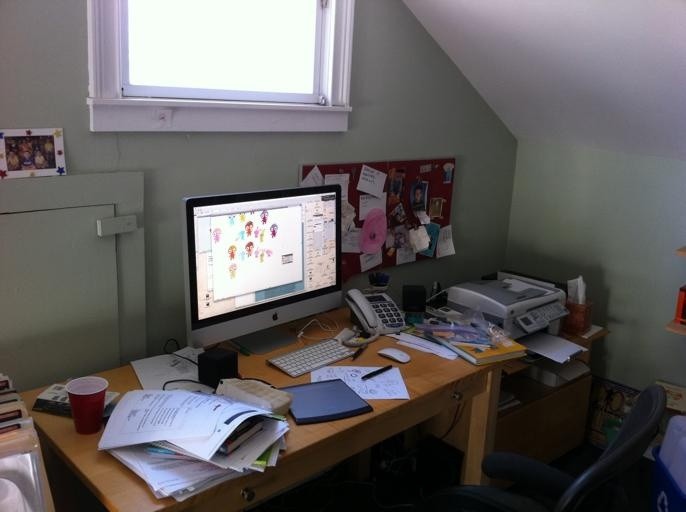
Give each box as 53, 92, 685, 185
345, 288, 406, 335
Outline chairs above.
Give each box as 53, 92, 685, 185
426, 382, 667, 512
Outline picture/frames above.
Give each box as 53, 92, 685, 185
0, 128, 68, 180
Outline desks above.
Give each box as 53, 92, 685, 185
17, 306, 506, 512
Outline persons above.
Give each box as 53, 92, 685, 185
5, 136, 55, 171
412, 185, 425, 209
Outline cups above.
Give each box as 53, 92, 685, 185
64, 377, 110, 434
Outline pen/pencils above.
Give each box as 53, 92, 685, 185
228, 340, 250, 357
351, 343, 368, 361
361, 365, 392, 380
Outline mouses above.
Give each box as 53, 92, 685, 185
376, 348, 411, 363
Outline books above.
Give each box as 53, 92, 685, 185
498, 395, 515, 405
96, 377, 292, 502
498, 400, 521, 411
0, 371, 57, 512
397, 319, 459, 356
428, 320, 529, 365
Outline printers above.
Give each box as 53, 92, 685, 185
445, 274, 590, 366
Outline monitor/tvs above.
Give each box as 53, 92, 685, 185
180, 183, 343, 356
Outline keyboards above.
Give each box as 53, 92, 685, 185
265, 338, 355, 378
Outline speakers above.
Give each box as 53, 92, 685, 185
197, 347, 239, 389
402, 284, 427, 312
